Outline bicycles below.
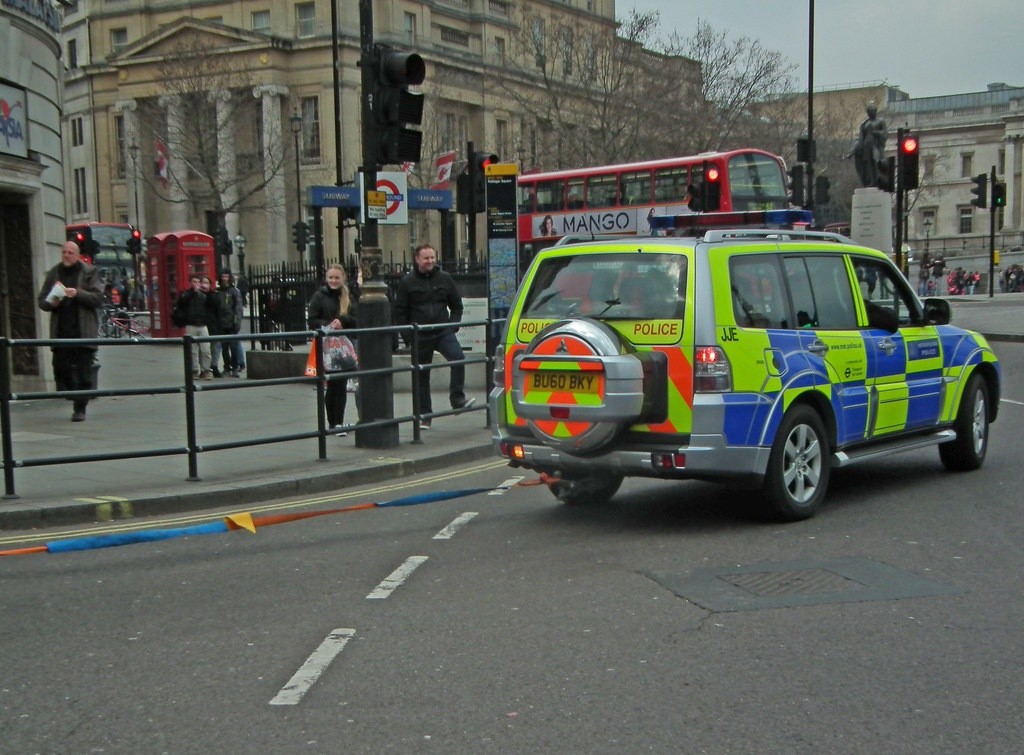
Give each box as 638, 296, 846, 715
95, 294, 154, 338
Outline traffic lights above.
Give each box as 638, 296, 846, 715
993, 182, 1007, 208
73, 230, 87, 256
292, 221, 302, 251
785, 165, 804, 207
900, 133, 919, 191
815, 175, 831, 206
471, 150, 500, 213
300, 222, 311, 252
131, 229, 143, 254
369, 40, 426, 168
126, 238, 130, 254
970, 173, 987, 209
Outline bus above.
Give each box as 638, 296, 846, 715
516, 148, 797, 320
65, 220, 146, 318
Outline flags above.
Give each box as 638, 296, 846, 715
156, 139, 168, 188
401, 161, 416, 177
432, 152, 457, 190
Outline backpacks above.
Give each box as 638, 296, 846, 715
170, 290, 187, 328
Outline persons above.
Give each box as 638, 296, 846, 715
525, 194, 534, 211
917, 249, 945, 296
236, 271, 249, 304
307, 264, 353, 437
539, 215, 556, 236
999, 264, 1024, 293
180, 268, 245, 380
39, 242, 103, 422
854, 105, 888, 188
864, 299, 898, 334
105, 284, 112, 302
855, 267, 890, 299
120, 278, 130, 312
947, 267, 980, 294
641, 208, 665, 237
395, 244, 475, 428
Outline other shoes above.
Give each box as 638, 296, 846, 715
205, 372, 213, 380
334, 424, 346, 436
420, 420, 431, 430
71, 410, 84, 421
221, 367, 232, 376
344, 423, 355, 430
193, 370, 199, 380
230, 363, 245, 374
452, 397, 476, 415
210, 367, 222, 378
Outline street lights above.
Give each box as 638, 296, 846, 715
288, 112, 304, 281
233, 231, 249, 306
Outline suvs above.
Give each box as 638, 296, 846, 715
488, 226, 1003, 524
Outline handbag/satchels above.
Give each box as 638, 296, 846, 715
323, 325, 358, 371
305, 338, 328, 387
